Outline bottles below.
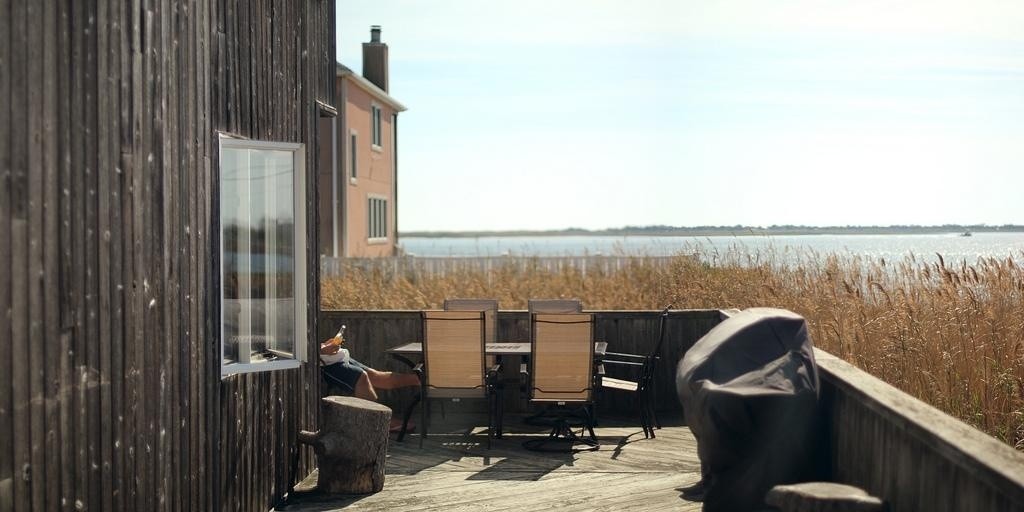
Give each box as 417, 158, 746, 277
332, 324, 346, 354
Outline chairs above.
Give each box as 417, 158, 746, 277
526, 295, 595, 426
442, 298, 501, 436
411, 310, 500, 451
592, 302, 672, 439
518, 311, 601, 452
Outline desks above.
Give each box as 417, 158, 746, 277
382, 341, 610, 448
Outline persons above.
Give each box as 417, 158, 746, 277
320, 338, 421, 401
